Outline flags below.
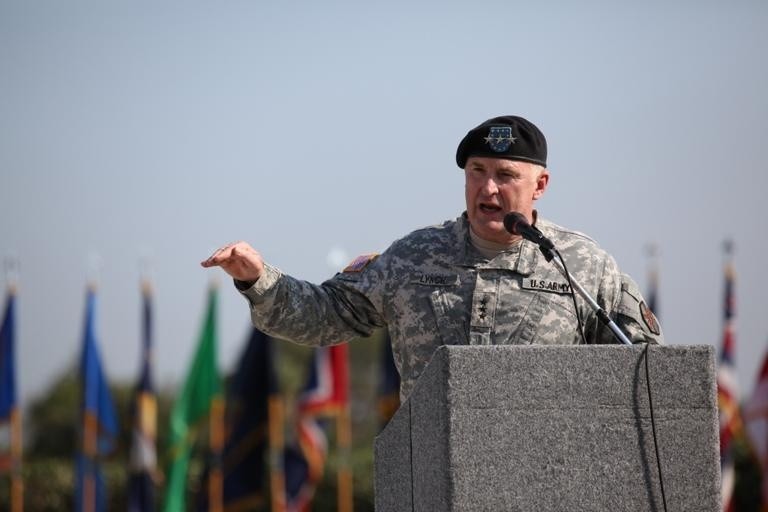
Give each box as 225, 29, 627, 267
649, 267, 768, 510
74, 285, 399, 511
0, 289, 21, 426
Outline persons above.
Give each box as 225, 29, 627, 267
199, 114, 661, 405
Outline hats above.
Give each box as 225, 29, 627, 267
456, 115, 548, 170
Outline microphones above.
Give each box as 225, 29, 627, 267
503, 211, 555, 251
